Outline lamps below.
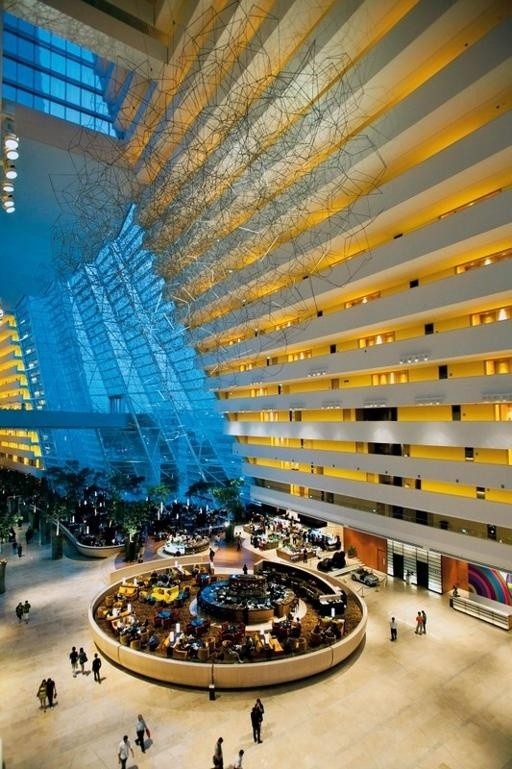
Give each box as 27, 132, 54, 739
0, 117, 20, 214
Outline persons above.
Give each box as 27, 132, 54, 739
254, 698, 264, 725
116, 735, 136, 769
92, 653, 102, 684
251, 707, 263, 744
133, 713, 150, 754
70, 647, 80, 678
11, 539, 18, 556
44, 678, 57, 710
22, 601, 32, 624
389, 617, 397, 642
15, 602, 25, 624
415, 612, 424, 635
233, 749, 245, 769
36, 680, 49, 713
99, 510, 342, 663
421, 610, 426, 633
8, 518, 37, 545
77, 647, 88, 676
210, 736, 225, 769
17, 543, 23, 558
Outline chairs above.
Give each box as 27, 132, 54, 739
97, 563, 345, 660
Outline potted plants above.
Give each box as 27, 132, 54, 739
348, 546, 356, 558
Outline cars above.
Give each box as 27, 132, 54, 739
352, 569, 379, 586
